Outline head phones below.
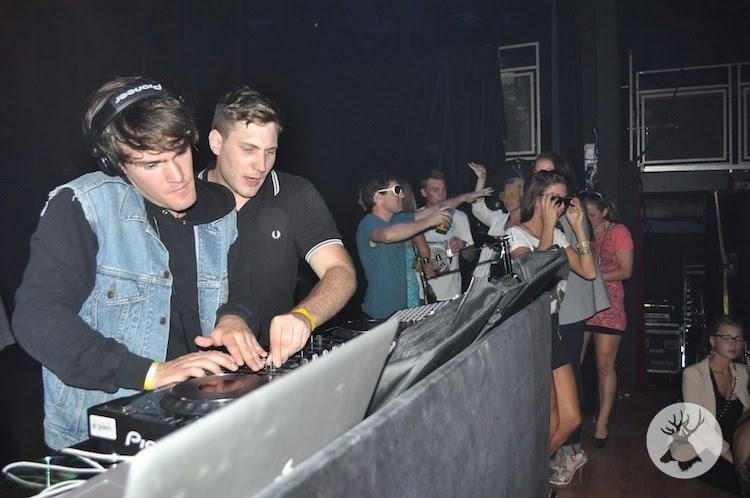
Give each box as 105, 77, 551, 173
89, 80, 201, 177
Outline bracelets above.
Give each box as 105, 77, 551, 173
464, 241, 468, 248
577, 240, 593, 257
142, 361, 159, 393
291, 306, 317, 331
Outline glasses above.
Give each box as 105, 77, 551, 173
377, 184, 404, 195
540, 193, 571, 208
713, 334, 745, 345
498, 191, 521, 201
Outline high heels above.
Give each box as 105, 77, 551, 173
592, 427, 610, 448
547, 449, 590, 486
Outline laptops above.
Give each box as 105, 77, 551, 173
52, 316, 401, 496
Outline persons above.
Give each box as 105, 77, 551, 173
190, 85, 360, 371
682, 314, 750, 491
532, 152, 561, 174
578, 190, 635, 448
550, 211, 610, 487
733, 379, 749, 498
414, 170, 475, 301
505, 169, 597, 498
355, 172, 494, 320
11, 76, 267, 454
468, 162, 525, 279
